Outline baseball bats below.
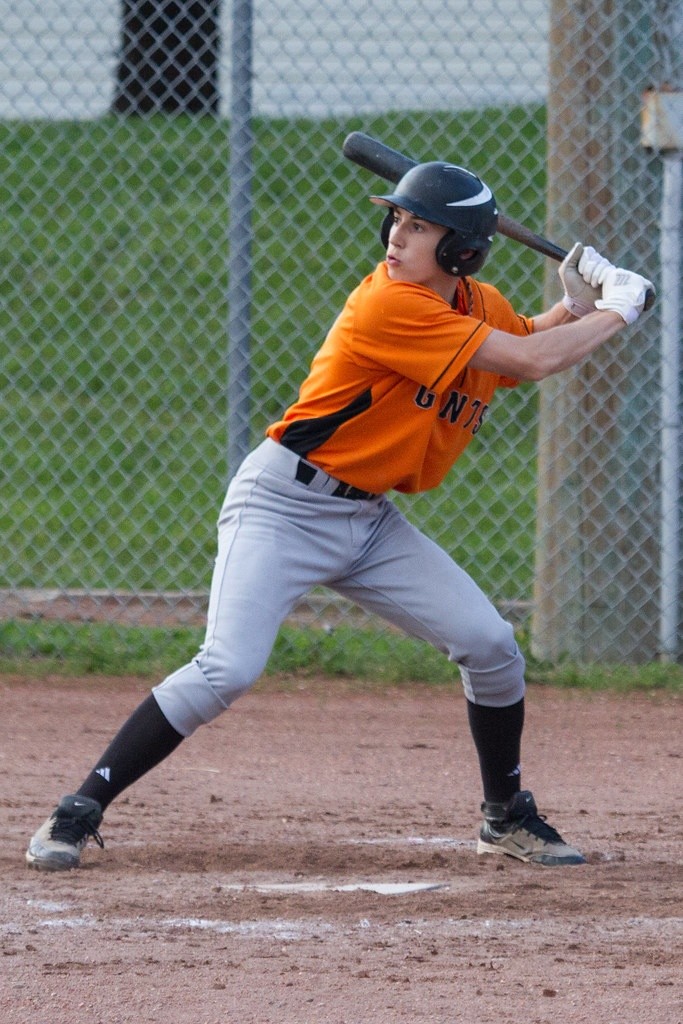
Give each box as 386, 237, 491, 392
342, 130, 656, 312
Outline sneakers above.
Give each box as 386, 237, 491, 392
476, 790, 589, 869
26, 795, 104, 872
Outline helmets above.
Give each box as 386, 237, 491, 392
368, 161, 498, 277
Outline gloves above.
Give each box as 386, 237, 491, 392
595, 268, 656, 326
557, 243, 615, 318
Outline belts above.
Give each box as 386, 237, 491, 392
294, 460, 380, 501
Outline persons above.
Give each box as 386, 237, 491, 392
27, 160, 654, 872
